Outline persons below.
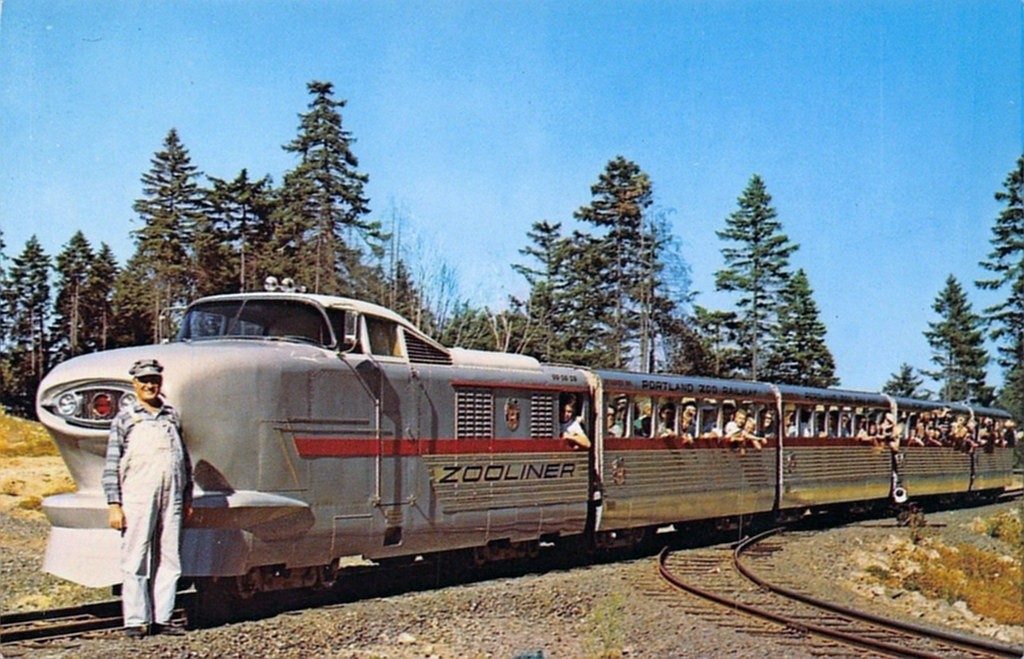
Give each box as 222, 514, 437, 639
102, 359, 194, 641
559, 393, 1018, 450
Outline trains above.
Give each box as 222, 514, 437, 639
43, 277, 1017, 622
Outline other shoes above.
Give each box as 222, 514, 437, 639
125, 626, 149, 639
150, 622, 186, 636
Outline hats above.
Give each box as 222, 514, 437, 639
129, 359, 163, 377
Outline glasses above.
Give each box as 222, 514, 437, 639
138, 375, 161, 384
